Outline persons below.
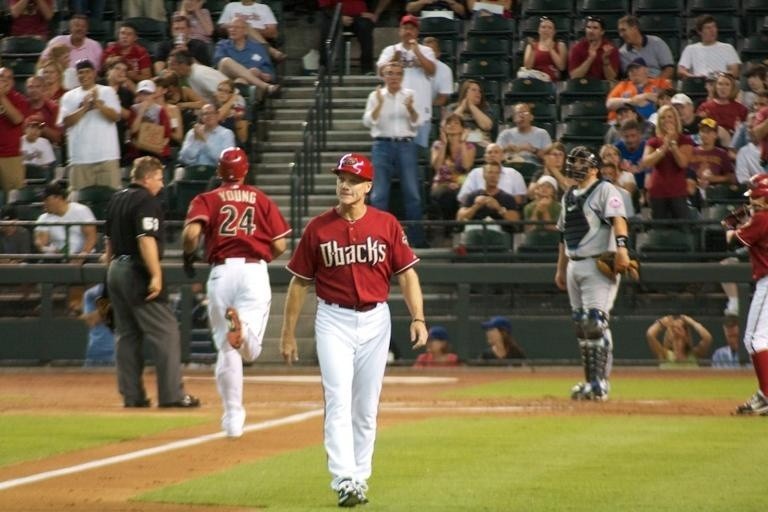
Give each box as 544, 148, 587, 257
101, 155, 200, 410
711, 316, 754, 369
278, 152, 429, 509
645, 312, 713, 369
81, 277, 118, 371
183, 146, 293, 440
553, 146, 632, 400
0, 1, 767, 322
408, 326, 462, 369
723, 172, 768, 416
477, 318, 529, 367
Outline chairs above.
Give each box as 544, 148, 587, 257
0, 1, 295, 256
406, 1, 768, 253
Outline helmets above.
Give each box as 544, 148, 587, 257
744, 174, 768, 197
565, 146, 603, 181
217, 147, 250, 182
332, 154, 374, 181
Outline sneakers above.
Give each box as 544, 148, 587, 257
227, 428, 243, 438
337, 479, 368, 506
736, 390, 768, 413
226, 308, 244, 349
572, 385, 608, 401
161, 394, 200, 407
125, 399, 149, 408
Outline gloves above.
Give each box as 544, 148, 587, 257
182, 250, 198, 277
721, 205, 750, 230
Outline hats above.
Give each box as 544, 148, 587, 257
427, 326, 448, 340
76, 59, 93, 71
625, 58, 647, 71
401, 16, 420, 29
136, 79, 156, 93
482, 318, 512, 333
537, 175, 559, 193
21, 115, 46, 129
671, 93, 692, 105
34, 183, 65, 200
698, 119, 719, 131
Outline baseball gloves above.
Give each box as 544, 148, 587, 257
598, 251, 640, 282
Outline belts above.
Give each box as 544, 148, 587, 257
211, 257, 262, 266
110, 254, 135, 262
569, 254, 601, 261
325, 300, 378, 312
373, 137, 413, 143
